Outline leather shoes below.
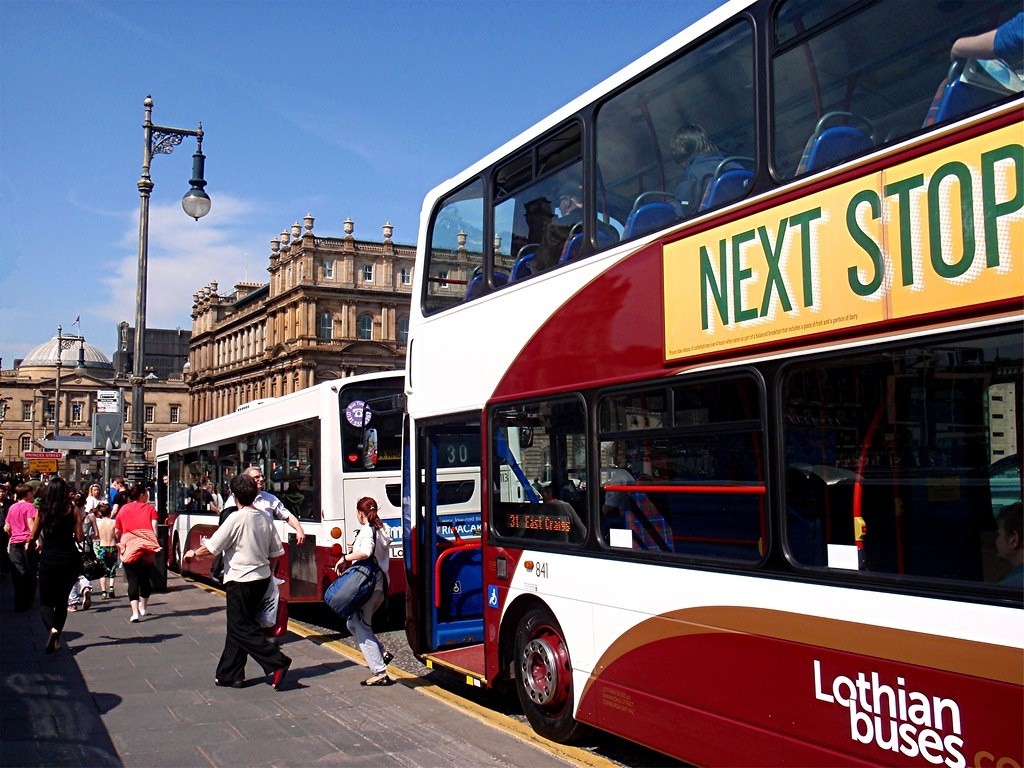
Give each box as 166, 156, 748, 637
361, 675, 390, 686
384, 653, 394, 665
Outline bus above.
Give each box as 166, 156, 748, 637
154, 371, 524, 608
404, 0, 1024, 768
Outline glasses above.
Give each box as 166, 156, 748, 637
254, 475, 266, 481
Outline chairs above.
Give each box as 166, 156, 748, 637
461, 57, 1024, 303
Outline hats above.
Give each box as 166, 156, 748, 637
556, 181, 584, 204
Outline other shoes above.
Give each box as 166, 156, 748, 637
214, 677, 243, 688
109, 588, 115, 598
45, 628, 61, 654
138, 601, 147, 616
272, 656, 292, 689
266, 636, 278, 644
129, 614, 139, 622
100, 593, 107, 598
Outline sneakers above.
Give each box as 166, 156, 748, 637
82, 588, 93, 610
67, 604, 77, 612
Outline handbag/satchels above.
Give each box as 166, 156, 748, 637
261, 596, 288, 637
71, 537, 105, 581
255, 574, 284, 629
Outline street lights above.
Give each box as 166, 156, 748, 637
129, 94, 213, 493
54, 323, 87, 438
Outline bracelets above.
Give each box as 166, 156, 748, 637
343, 555, 347, 562
194, 550, 196, 556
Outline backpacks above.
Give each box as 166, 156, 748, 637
323, 526, 388, 619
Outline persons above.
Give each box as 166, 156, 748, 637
163, 474, 168, 486
27, 477, 85, 654
185, 474, 292, 689
335, 497, 395, 686
223, 466, 306, 545
667, 122, 744, 217
115, 484, 163, 622
0, 468, 130, 612
188, 477, 232, 516
532, 478, 542, 493
996, 503, 1024, 585
950, 11, 1024, 61
536, 188, 602, 272
516, 485, 587, 543
603, 439, 639, 517
